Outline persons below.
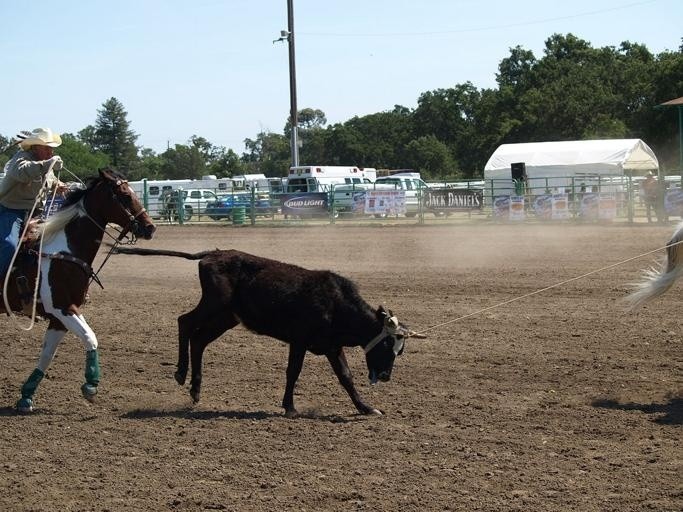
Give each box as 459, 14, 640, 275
579, 186, 586, 193
642, 170, 659, 223
591, 185, 598, 192
0, 126, 70, 291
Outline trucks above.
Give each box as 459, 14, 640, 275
123, 166, 444, 221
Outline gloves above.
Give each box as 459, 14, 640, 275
48, 154, 63, 171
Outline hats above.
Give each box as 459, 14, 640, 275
21, 127, 63, 151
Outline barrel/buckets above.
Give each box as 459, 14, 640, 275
232, 206, 245, 224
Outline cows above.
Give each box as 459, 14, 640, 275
95, 239, 428, 419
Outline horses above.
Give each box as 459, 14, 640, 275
612, 223, 683, 317
0, 163, 160, 413
